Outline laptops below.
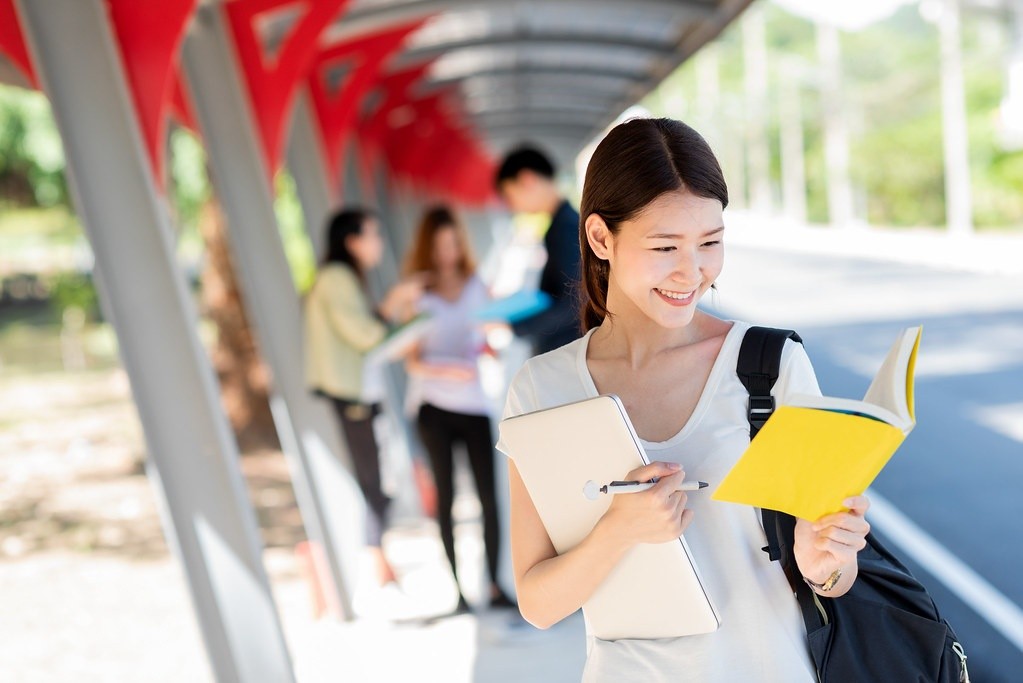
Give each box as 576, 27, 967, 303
500, 393, 722, 639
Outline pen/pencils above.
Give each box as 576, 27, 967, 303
600, 480, 709, 493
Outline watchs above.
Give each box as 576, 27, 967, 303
804, 564, 842, 592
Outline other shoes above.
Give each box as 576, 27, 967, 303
453, 592, 473, 617
486, 586, 518, 609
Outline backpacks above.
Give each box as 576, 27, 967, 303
736, 326, 973, 683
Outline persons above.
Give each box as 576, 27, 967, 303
300, 143, 587, 627
496, 118, 871, 683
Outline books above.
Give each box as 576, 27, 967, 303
710, 325, 924, 523
